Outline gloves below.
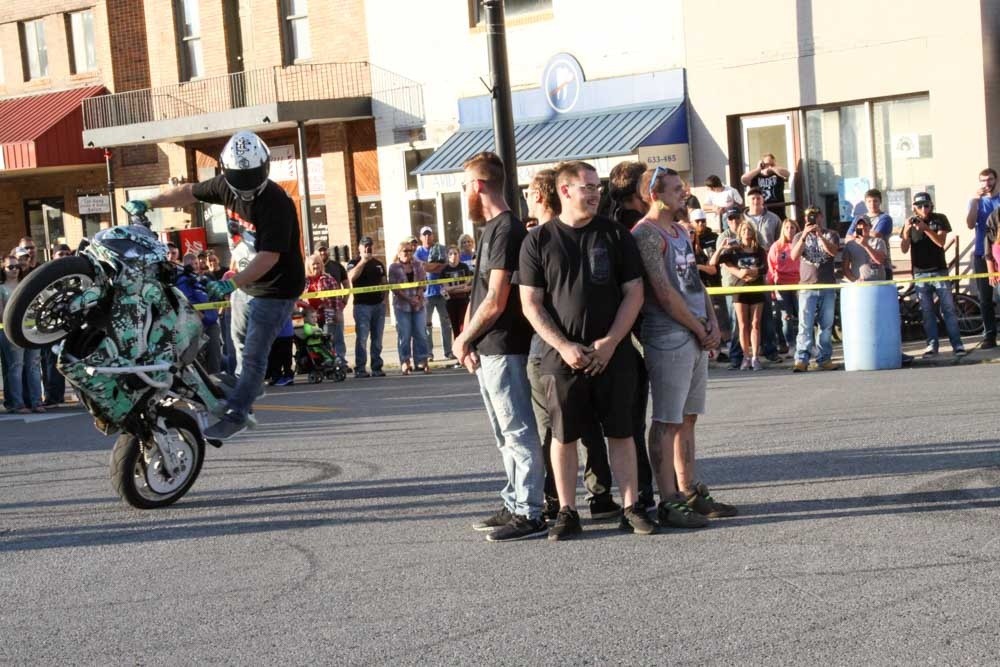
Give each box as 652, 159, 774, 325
126, 199, 152, 215
206, 278, 237, 301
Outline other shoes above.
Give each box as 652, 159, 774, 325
473, 479, 737, 542
264, 359, 429, 385
712, 344, 1000, 373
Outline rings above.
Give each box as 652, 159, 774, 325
597, 362, 603, 368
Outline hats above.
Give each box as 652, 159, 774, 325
11, 247, 30, 258
912, 192, 931, 205
727, 207, 740, 216
315, 242, 326, 250
360, 237, 372, 245
855, 214, 871, 225
420, 226, 432, 234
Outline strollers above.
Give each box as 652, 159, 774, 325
291, 311, 348, 383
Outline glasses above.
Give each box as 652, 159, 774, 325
522, 189, 537, 201
461, 179, 485, 191
566, 183, 605, 193
918, 204, 929, 208
58, 252, 70, 256
728, 214, 740, 220
403, 250, 412, 253
650, 166, 666, 193
695, 219, 706, 223
6, 264, 20, 270
25, 246, 35, 249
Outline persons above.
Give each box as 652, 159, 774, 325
450, 150, 734, 544
123, 131, 306, 441
0, 237, 90, 415
163, 224, 478, 387
673, 152, 1000, 372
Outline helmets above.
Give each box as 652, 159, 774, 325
219, 132, 271, 201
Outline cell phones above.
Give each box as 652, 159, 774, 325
366, 247, 372, 253
856, 228, 862, 238
727, 239, 740, 247
440, 290, 451, 301
808, 214, 816, 225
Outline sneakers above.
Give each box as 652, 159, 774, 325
203, 409, 248, 440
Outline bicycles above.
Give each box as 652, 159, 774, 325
898, 280, 984, 335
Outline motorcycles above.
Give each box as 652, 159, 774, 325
3, 206, 257, 511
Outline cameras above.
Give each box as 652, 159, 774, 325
910, 217, 919, 224
